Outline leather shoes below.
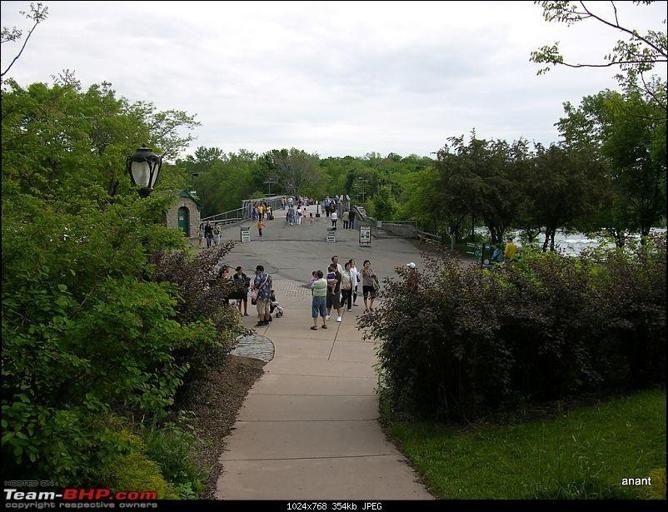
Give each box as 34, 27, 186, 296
364, 308, 374, 314
240, 313, 248, 317
353, 304, 358, 306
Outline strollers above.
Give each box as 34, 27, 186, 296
269, 300, 283, 319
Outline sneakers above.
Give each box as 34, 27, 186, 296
256, 316, 272, 326
326, 314, 331, 319
349, 309, 351, 312
336, 317, 341, 321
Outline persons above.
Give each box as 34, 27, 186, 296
491, 238, 516, 263
406, 262, 415, 270
310, 256, 380, 331
252, 195, 355, 236
216, 265, 275, 327
199, 221, 223, 249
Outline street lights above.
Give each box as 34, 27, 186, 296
191, 174, 199, 185
126, 143, 162, 245
268, 157, 275, 195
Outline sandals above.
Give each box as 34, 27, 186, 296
322, 325, 327, 329
311, 326, 318, 330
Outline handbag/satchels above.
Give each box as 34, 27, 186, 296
250, 291, 257, 300
